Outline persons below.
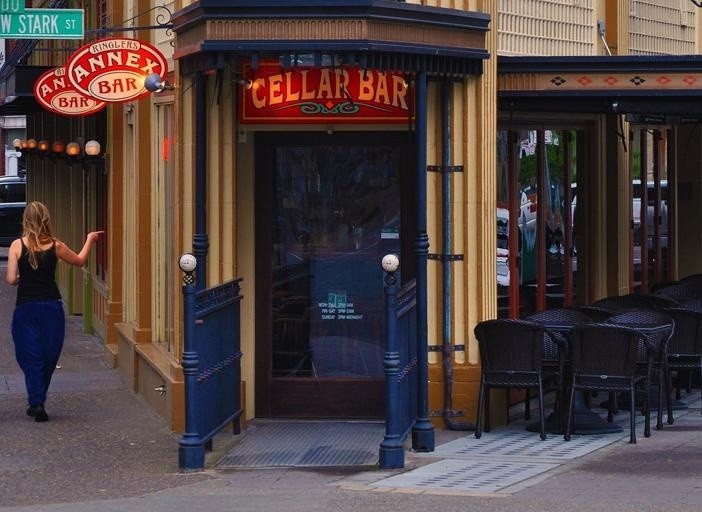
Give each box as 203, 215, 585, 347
6, 201, 105, 422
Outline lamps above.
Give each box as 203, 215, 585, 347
13, 138, 110, 177
144, 74, 176, 93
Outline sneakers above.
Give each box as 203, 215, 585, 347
26, 405, 49, 422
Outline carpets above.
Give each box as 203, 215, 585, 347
216, 423, 386, 468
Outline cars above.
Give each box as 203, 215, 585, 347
0, 176, 26, 258
497, 179, 668, 289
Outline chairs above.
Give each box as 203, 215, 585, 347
474, 273, 702, 445
